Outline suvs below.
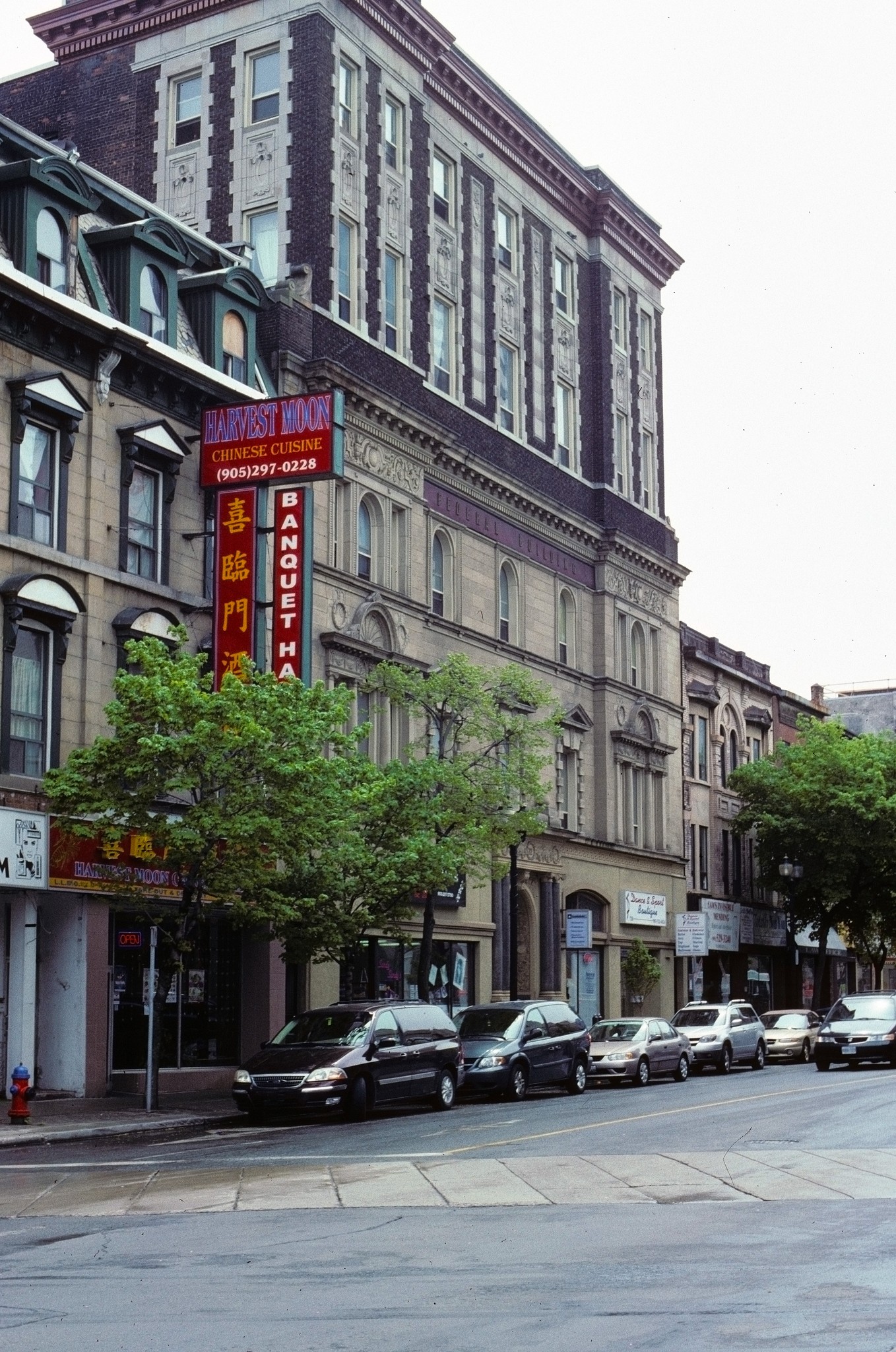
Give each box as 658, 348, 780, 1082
451, 1000, 592, 1102
232, 999, 464, 1123
813, 990, 896, 1072
668, 999, 768, 1075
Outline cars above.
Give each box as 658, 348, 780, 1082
759, 1010, 823, 1064
586, 1016, 694, 1087
815, 1007, 832, 1022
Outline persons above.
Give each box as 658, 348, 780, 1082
454, 959, 462, 985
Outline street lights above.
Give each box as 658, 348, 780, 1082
779, 853, 804, 1010
491, 799, 530, 1002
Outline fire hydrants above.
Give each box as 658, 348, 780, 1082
7, 1063, 37, 1126
